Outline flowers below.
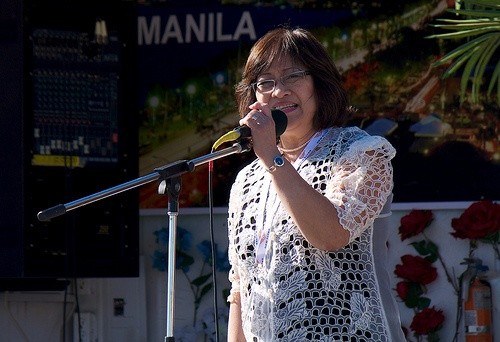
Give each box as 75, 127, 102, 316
151, 226, 230, 342
392, 200, 500, 342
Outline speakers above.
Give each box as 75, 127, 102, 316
1, 1, 142, 291
71, 279, 146, 342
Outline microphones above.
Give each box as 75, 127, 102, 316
235, 111, 288, 144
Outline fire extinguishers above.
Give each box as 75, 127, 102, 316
463, 258, 492, 342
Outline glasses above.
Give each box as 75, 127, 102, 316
250, 69, 312, 94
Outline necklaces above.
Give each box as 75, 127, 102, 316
277, 142, 310, 152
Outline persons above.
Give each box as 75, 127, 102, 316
228, 26, 406, 342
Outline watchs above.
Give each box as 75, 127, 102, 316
267, 155, 284, 173
